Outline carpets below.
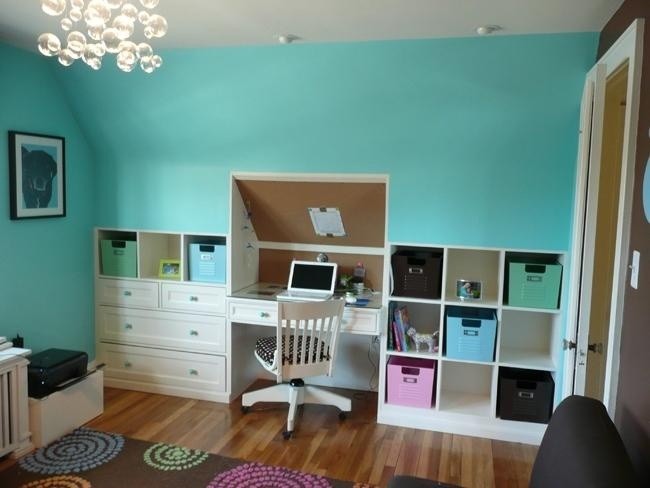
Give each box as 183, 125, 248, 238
0, 427, 378, 488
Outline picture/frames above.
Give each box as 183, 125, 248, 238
7, 129, 67, 221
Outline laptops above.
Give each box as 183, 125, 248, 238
276, 260, 338, 302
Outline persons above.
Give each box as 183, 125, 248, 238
459, 282, 473, 299
467, 289, 473, 299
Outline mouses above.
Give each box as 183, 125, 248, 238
346, 296, 356, 304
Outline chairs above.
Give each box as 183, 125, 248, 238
239, 296, 352, 441
386, 394, 643, 488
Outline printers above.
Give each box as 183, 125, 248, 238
24, 348, 106, 399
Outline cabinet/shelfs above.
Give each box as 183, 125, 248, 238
92, 278, 231, 406
378, 240, 570, 448
93, 225, 226, 286
228, 280, 391, 336
29, 368, 105, 449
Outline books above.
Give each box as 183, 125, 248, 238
393, 322, 401, 352
0, 336, 7, 343
394, 305, 412, 351
0, 343, 13, 351
388, 302, 393, 350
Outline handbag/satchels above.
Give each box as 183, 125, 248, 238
24, 349, 88, 398
